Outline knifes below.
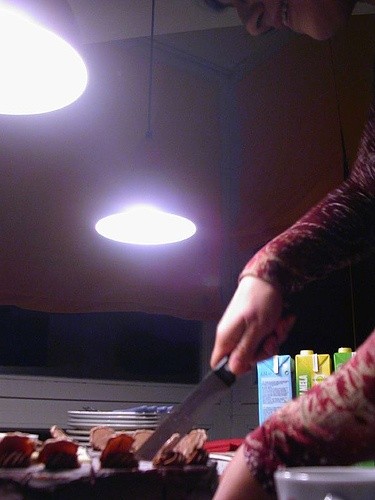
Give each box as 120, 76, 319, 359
134, 327, 277, 461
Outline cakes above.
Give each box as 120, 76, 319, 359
0, 425, 218, 500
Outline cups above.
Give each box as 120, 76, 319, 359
273, 465, 375, 500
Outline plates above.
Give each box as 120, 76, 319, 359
65, 410, 161, 448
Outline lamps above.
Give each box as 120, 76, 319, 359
0, 0, 89, 115
94, 0, 197, 245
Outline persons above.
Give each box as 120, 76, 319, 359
197, 0, 375, 500
